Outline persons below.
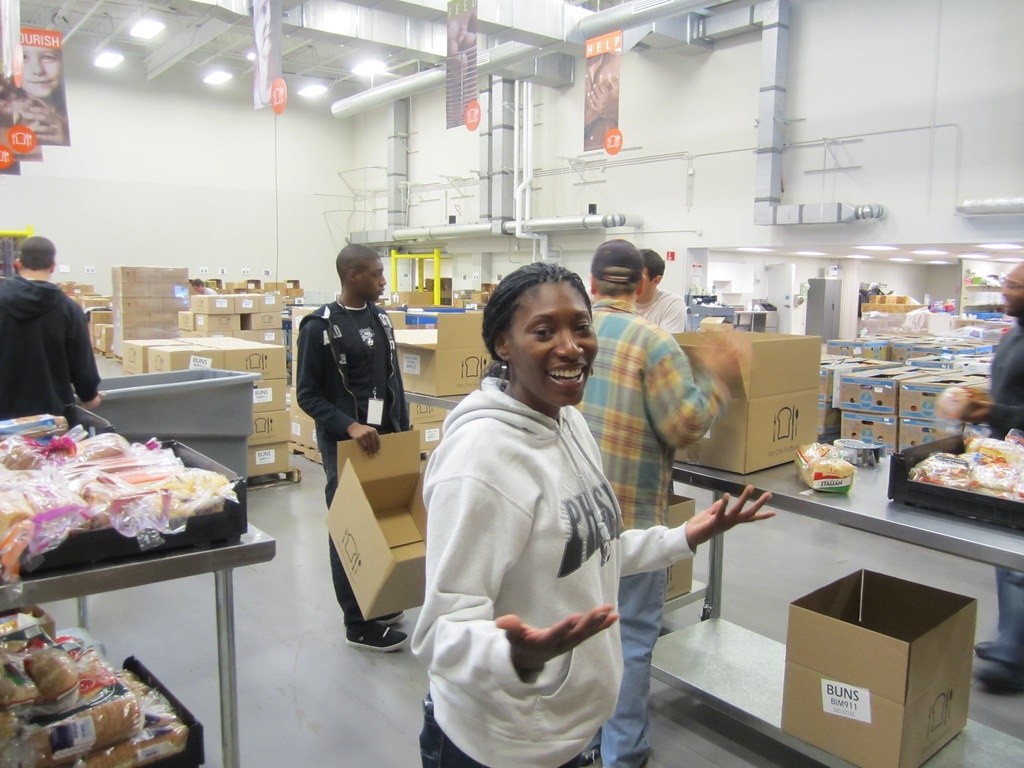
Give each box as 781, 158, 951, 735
956, 260, 1024, 695
637, 247, 688, 333
298, 243, 412, 652
253, 0, 284, 110
14, 47, 72, 144
574, 238, 742, 768
2, 236, 103, 421
191, 278, 217, 295
412, 263, 777, 767
585, 51, 619, 151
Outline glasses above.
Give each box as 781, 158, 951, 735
997, 277, 1024, 291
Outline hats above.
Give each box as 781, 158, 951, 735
590, 238, 644, 285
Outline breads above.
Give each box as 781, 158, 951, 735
795, 385, 1024, 504
0, 411, 236, 768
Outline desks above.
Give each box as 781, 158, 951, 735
660, 457, 1022, 767
0, 517, 276, 768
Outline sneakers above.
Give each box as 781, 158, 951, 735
369, 611, 404, 625
345, 627, 407, 652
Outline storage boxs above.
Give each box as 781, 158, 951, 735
62, 263, 1014, 622
780, 568, 977, 768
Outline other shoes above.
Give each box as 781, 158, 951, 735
578, 744, 601, 767
976, 668, 1024, 693
974, 641, 1024, 673
